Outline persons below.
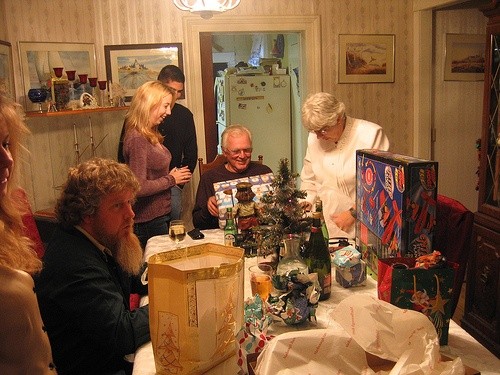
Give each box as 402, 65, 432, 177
0, 92, 56, 375
298, 91, 390, 241
118, 64, 198, 221
193, 123, 273, 230
123, 80, 193, 253
34, 157, 151, 375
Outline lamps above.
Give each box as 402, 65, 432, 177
173, 0, 240, 20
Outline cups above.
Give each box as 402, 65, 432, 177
248, 264, 273, 303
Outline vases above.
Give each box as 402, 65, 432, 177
276, 234, 309, 277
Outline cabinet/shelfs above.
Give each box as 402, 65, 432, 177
461, 0, 500, 359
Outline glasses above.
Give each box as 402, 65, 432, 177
313, 124, 330, 135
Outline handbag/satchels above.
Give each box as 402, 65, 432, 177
141, 242, 245, 375
378, 256, 459, 347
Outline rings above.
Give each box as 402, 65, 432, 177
183, 176, 185, 179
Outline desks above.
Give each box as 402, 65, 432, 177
131, 218, 500, 375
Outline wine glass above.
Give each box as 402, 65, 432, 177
168, 220, 185, 249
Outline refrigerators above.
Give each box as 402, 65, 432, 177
215, 75, 292, 175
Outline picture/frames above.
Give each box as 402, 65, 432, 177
337, 34, 395, 85
104, 43, 185, 104
443, 32, 486, 81
0, 40, 16, 108
17, 42, 97, 114
212, 52, 235, 85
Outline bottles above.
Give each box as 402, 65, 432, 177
223, 208, 237, 247
315, 201, 329, 249
277, 239, 308, 276
304, 212, 331, 301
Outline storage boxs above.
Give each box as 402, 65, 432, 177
213, 173, 278, 229
332, 245, 367, 288
355, 148, 438, 283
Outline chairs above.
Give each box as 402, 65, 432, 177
199, 155, 263, 181
437, 194, 475, 318
